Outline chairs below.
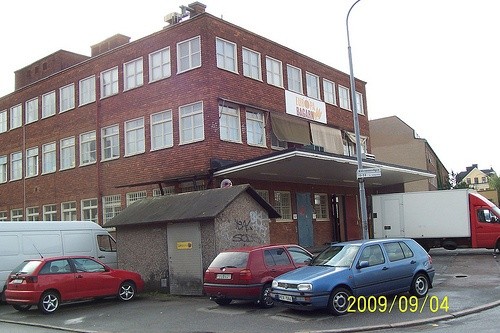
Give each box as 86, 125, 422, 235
51, 266, 59, 272
365, 247, 405, 267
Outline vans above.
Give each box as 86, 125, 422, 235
0, 219, 117, 305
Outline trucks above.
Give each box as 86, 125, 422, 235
367, 189, 500, 255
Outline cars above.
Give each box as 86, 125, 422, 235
3, 256, 144, 314
271, 236, 437, 316
204, 243, 316, 308
